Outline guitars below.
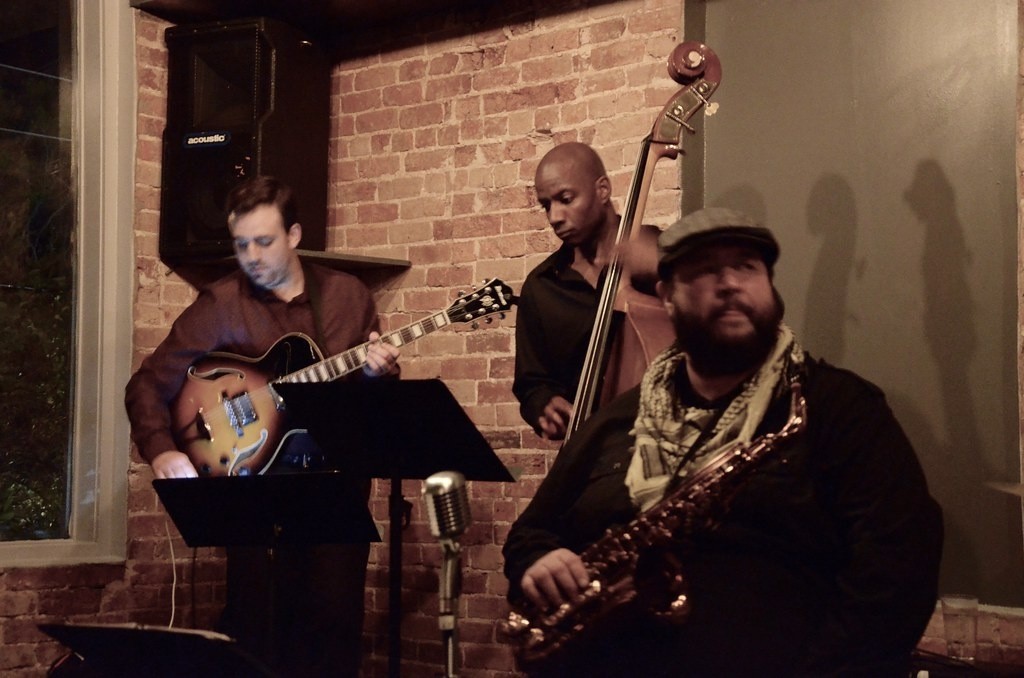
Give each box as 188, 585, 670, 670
170, 276, 519, 474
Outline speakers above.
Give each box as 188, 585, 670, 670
156, 18, 330, 264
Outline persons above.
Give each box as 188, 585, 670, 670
125, 174, 400, 678
512, 141, 678, 445
502, 207, 945, 678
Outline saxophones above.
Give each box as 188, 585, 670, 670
491, 359, 813, 669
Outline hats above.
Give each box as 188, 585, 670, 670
656, 207, 780, 268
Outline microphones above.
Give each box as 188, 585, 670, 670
420, 471, 472, 632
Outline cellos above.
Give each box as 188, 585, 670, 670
565, 39, 725, 446
